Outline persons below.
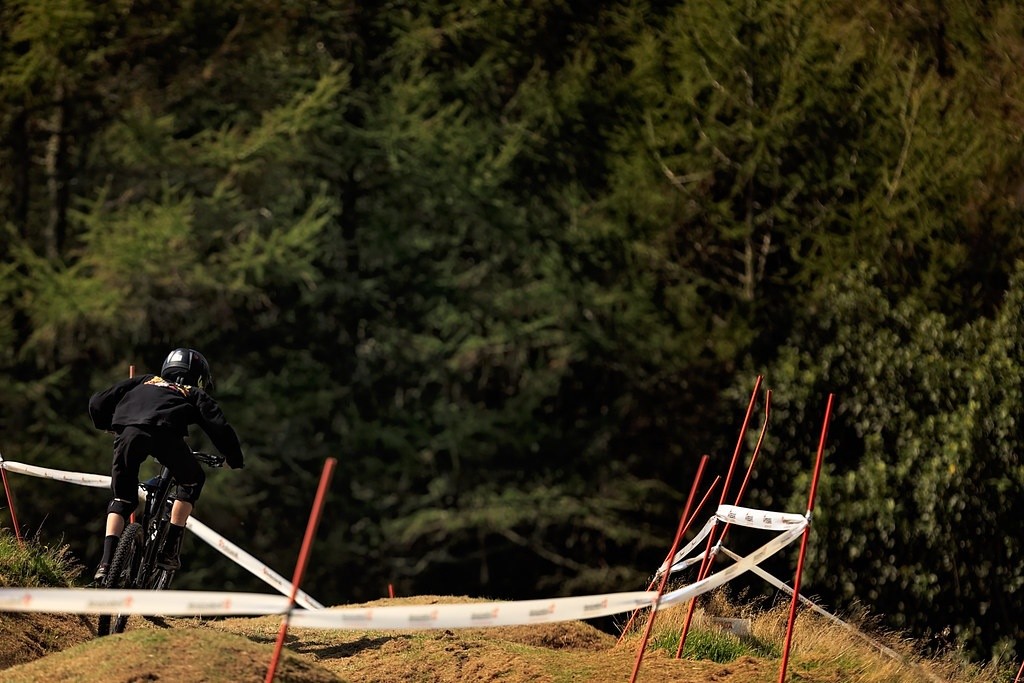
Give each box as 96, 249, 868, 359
90, 348, 246, 585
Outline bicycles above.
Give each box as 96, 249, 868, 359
95, 425, 246, 639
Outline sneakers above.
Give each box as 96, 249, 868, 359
94, 563, 110, 583
157, 552, 181, 571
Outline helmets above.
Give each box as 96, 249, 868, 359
161, 347, 214, 392
